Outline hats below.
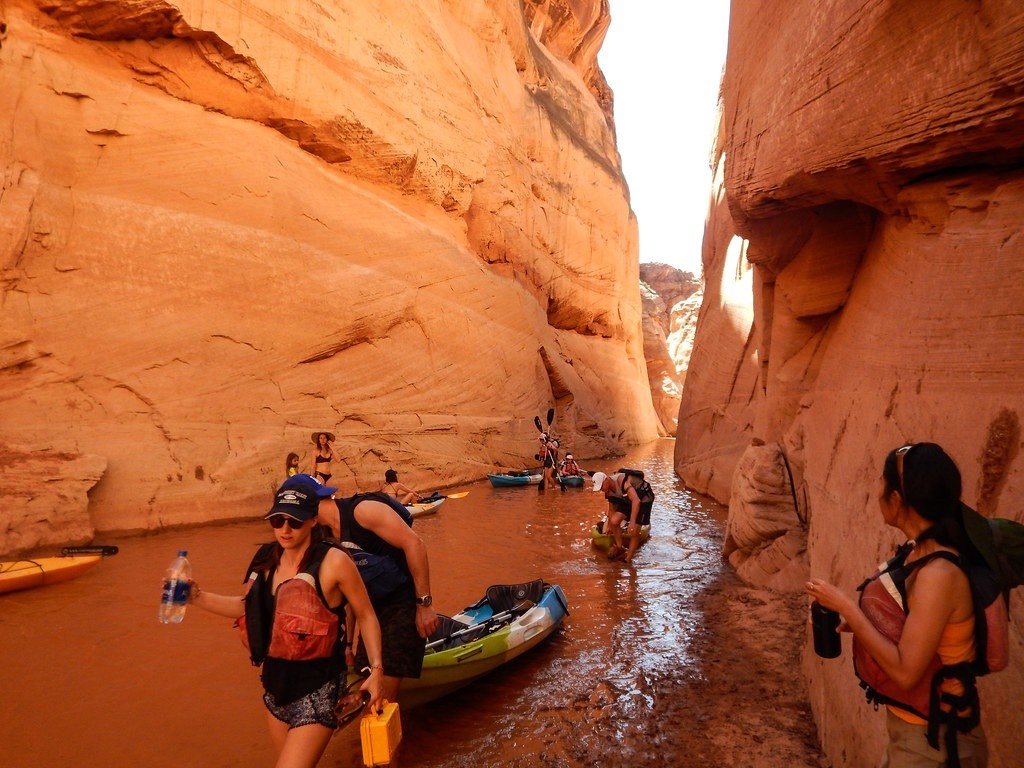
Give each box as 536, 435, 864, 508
311, 432, 336, 444
592, 472, 607, 491
539, 434, 546, 439
282, 474, 337, 496
566, 455, 574, 460
384, 469, 397, 477
264, 483, 319, 521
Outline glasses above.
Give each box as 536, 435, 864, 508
896, 443, 915, 493
270, 514, 304, 529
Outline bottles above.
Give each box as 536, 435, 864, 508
158, 550, 192, 624
811, 599, 842, 659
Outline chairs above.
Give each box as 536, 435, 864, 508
428, 614, 482, 652
486, 578, 544, 613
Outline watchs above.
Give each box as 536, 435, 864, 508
415, 594, 433, 608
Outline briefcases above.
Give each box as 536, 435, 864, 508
360, 701, 402, 766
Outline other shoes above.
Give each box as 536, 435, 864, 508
607, 544, 624, 558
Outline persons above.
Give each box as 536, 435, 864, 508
557, 452, 580, 476
161, 483, 385, 768
592, 472, 655, 564
538, 432, 559, 490
310, 432, 335, 488
804, 442, 1024, 768
562, 455, 581, 478
283, 474, 439, 768
286, 452, 300, 478
382, 470, 423, 506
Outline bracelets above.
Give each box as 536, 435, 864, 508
371, 666, 384, 672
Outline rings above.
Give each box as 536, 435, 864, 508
631, 529, 633, 531
810, 583, 814, 591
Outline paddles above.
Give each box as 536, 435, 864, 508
402, 503, 435, 508
538, 408, 555, 491
424, 599, 535, 649
535, 415, 567, 493
417, 491, 470, 501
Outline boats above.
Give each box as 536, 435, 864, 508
404, 491, 470, 516
346, 578, 568, 693
591, 521, 651, 548
487, 470, 585, 487
0, 545, 119, 593
